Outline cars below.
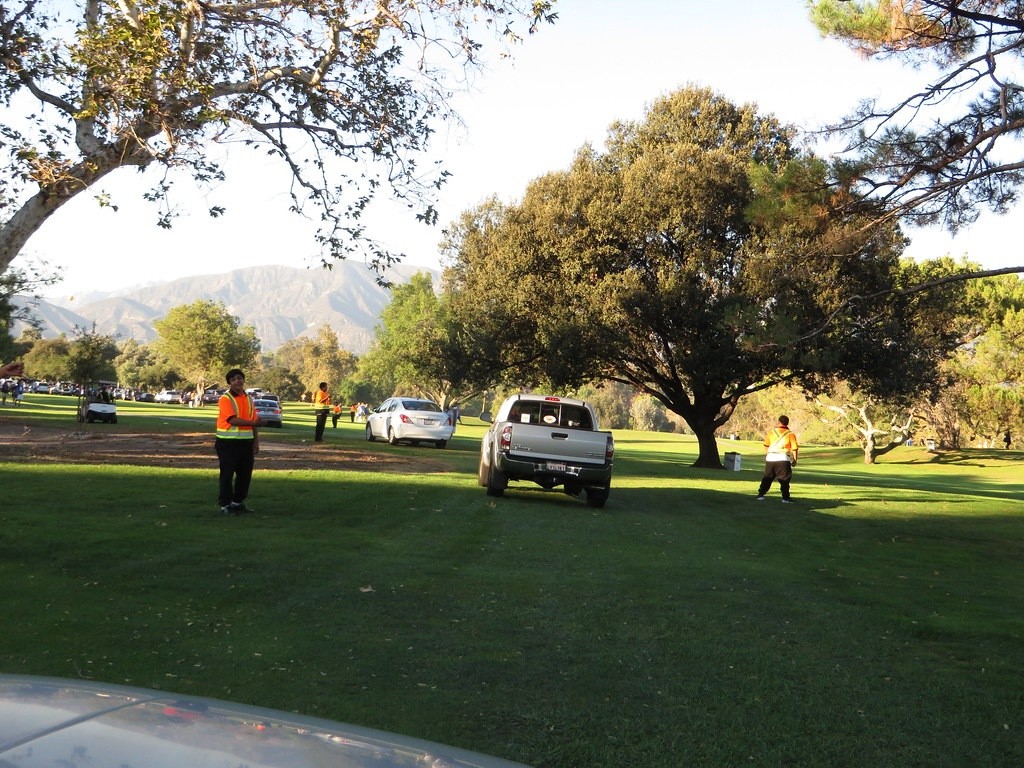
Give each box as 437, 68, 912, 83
252, 400, 283, 427
1, 375, 281, 403
364, 396, 454, 450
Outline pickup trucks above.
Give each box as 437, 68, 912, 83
477, 393, 615, 509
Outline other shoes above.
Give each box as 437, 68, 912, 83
757, 496, 765, 501
221, 505, 238, 515
231, 503, 253, 513
782, 498, 796, 503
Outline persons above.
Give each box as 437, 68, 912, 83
755, 415, 799, 504
0, 378, 24, 406
0, 362, 25, 378
351, 402, 370, 423
315, 382, 330, 443
1003, 431, 1012, 449
447, 397, 460, 434
48, 380, 68, 395
332, 401, 342, 428
98, 387, 110, 403
214, 369, 259, 515
183, 391, 205, 407
109, 387, 136, 404
735, 431, 740, 441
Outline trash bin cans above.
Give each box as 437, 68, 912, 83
723, 451, 742, 472
